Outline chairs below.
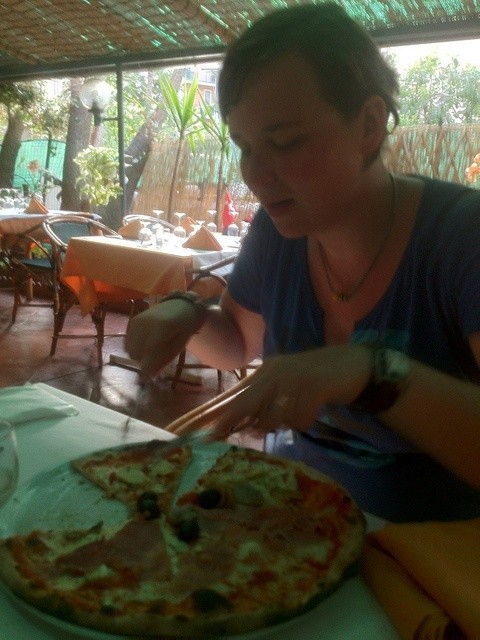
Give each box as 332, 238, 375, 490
170, 253, 263, 396
10, 224, 66, 324
125, 215, 174, 235
42, 215, 135, 371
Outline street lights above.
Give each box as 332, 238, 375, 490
78, 78, 127, 222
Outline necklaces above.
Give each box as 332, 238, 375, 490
318, 172, 398, 301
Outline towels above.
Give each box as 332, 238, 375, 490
118, 221, 144, 241
23, 197, 50, 215
360, 520, 480, 639
183, 227, 224, 251
181, 217, 197, 235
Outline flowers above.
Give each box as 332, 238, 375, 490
5, 158, 55, 196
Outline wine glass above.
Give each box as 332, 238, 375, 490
207, 210, 217, 232
13, 188, 23, 210
4, 188, 13, 209
196, 220, 205, 226
227, 211, 239, 236
173, 212, 186, 242
0, 419, 20, 510
152, 210, 164, 223
0, 188, 4, 208
139, 221, 152, 246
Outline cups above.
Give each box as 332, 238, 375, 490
189, 224, 201, 235
155, 230, 162, 248
164, 229, 170, 241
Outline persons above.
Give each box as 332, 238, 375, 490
124, 3, 480, 522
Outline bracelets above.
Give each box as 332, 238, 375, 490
355, 348, 413, 416
160, 288, 208, 334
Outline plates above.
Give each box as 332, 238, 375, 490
0, 438, 367, 640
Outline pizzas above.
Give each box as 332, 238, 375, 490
2, 439, 366, 636
